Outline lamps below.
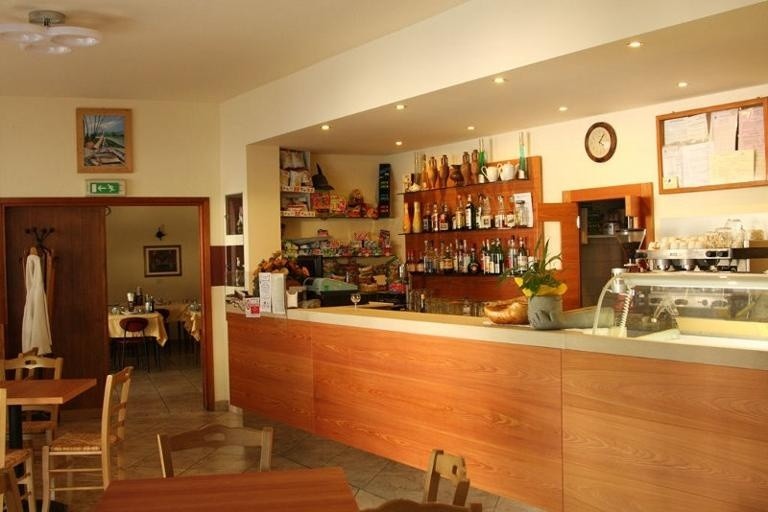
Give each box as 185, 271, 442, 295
0, 10, 101, 54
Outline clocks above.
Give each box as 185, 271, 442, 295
584, 122, 617, 162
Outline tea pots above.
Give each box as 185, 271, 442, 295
498, 162, 520, 182
480, 166, 501, 182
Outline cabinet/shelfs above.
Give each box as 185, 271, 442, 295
280, 214, 405, 311
397, 156, 543, 302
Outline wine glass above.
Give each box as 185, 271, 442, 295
350, 293, 361, 312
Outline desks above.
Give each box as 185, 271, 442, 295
2, 375, 96, 511
99, 465, 361, 511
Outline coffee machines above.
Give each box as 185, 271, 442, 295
645, 250, 768, 331
615, 227, 666, 330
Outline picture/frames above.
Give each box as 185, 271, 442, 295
144, 245, 181, 277
76, 108, 133, 173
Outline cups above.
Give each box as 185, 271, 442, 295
410, 288, 484, 317
647, 217, 766, 249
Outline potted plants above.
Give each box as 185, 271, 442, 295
497, 235, 567, 330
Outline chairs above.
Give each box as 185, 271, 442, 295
0, 388, 39, 512
156, 424, 275, 479
41, 366, 136, 511
362, 447, 483, 511
2, 347, 65, 440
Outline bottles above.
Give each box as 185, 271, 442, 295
611, 268, 626, 293
402, 193, 529, 234
111, 285, 200, 315
406, 237, 536, 277
